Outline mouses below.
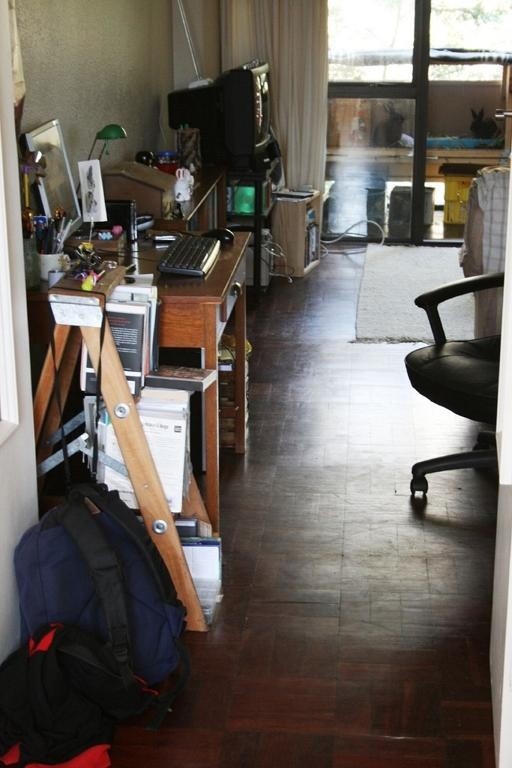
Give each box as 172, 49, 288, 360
201, 227, 234, 242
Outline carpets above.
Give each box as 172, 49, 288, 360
355, 242, 475, 343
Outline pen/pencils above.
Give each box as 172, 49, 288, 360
33, 204, 73, 254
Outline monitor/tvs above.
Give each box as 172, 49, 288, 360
19, 118, 83, 260
167, 62, 274, 171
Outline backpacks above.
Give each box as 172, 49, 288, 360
15, 480, 187, 731
0, 623, 120, 764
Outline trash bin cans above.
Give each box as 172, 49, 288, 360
218, 333, 252, 449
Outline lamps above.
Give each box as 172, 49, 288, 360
75, 124, 127, 194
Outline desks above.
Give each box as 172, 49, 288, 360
103, 158, 228, 231
39, 227, 253, 535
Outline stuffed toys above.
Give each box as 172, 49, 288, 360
173, 167, 195, 201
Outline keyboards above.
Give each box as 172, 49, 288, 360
156, 233, 221, 277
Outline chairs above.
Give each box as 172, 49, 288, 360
396, 272, 503, 499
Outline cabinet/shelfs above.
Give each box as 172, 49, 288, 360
228, 156, 282, 301
270, 189, 322, 278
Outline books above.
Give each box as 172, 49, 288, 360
80, 272, 224, 607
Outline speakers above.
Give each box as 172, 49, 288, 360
94, 198, 138, 243
